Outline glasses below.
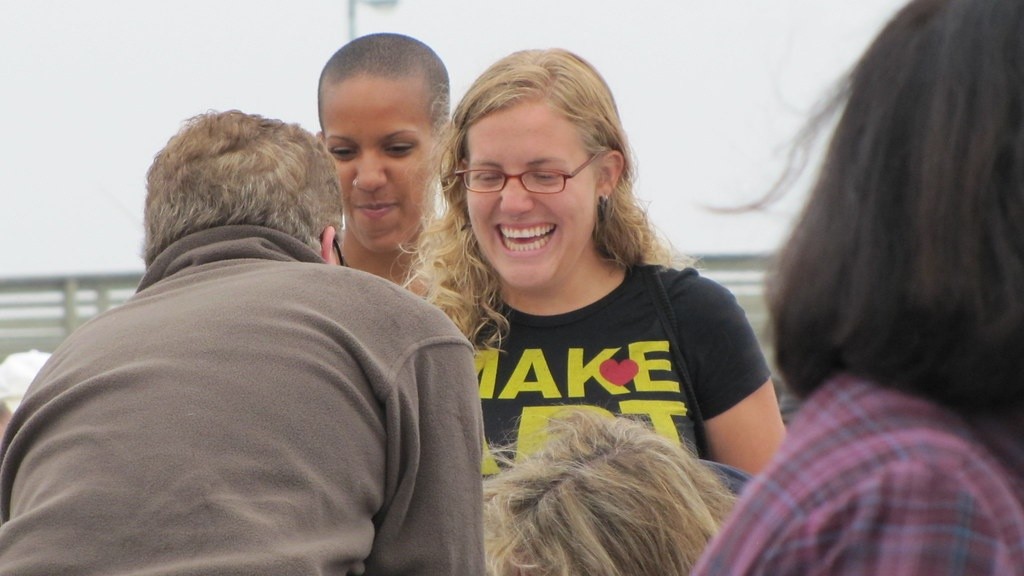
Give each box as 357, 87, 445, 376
454, 151, 600, 194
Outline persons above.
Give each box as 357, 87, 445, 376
404, 46, 791, 485
0, 105, 490, 576
684, 1, 1023, 576
304, 28, 459, 307
0, 340, 56, 439
473, 399, 754, 576
